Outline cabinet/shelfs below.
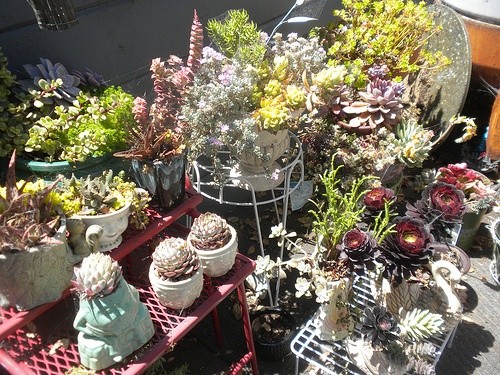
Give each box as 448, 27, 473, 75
0, 193, 256, 375
291, 268, 464, 375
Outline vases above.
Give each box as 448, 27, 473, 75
253, 307, 296, 360
388, 171, 403, 196
489, 218, 500, 286
427, 241, 471, 275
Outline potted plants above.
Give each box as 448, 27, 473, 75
56, 169, 152, 252
149, 237, 203, 309
268, 223, 356, 342
181, 9, 308, 191
187, 212, 237, 277
417, 163, 497, 251
0, 149, 76, 311
73, 252, 157, 371
0, 47, 136, 178
113, 122, 186, 209
